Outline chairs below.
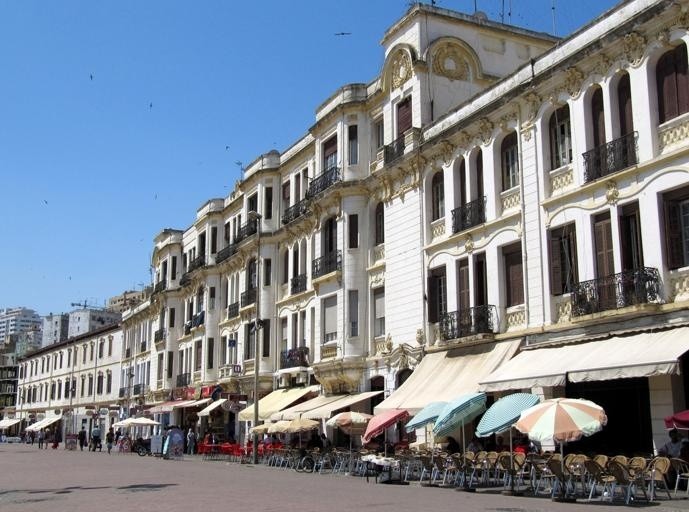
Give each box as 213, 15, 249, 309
197, 441, 689, 506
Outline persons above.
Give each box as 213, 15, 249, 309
19, 424, 133, 454
678, 433, 689, 474
650, 429, 682, 491
373, 440, 395, 458
263, 433, 331, 455
444, 435, 463, 455
162, 423, 221, 457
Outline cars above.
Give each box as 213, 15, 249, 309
133, 439, 151, 456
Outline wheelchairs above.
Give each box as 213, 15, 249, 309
294, 448, 318, 473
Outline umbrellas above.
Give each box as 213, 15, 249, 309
432, 392, 487, 459
112, 417, 136, 436
514, 396, 608, 472
404, 400, 452, 451
128, 416, 162, 440
363, 409, 409, 455
250, 411, 374, 446
663, 409, 688, 432
475, 392, 542, 470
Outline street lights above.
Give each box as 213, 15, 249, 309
246, 207, 261, 463
